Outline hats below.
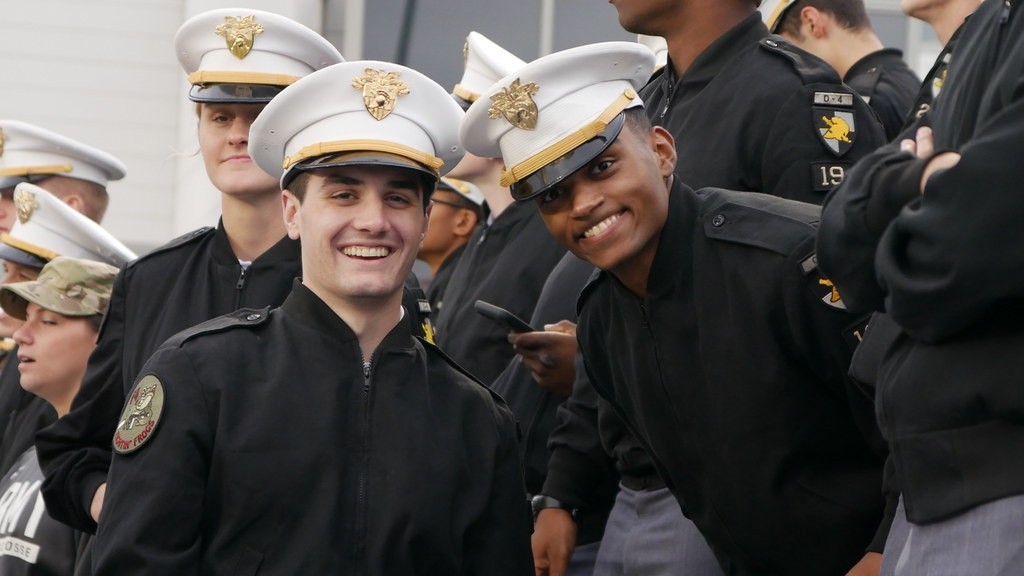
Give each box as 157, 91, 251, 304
0, 120, 126, 189
458, 41, 656, 203
247, 60, 466, 201
437, 176, 486, 206
0, 182, 138, 272
0, 256, 120, 321
756, 0, 795, 33
450, 31, 528, 111
174, 8, 346, 104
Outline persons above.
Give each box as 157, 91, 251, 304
0, 119, 135, 575
609, 0, 889, 207
35, 9, 436, 533
460, 41, 883, 576
418, 32, 619, 576
766, 1, 923, 137
818, 0, 1024, 576
86, 61, 541, 576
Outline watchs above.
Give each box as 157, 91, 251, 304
529, 494, 571, 522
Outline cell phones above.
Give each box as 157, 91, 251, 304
473, 299, 538, 334
